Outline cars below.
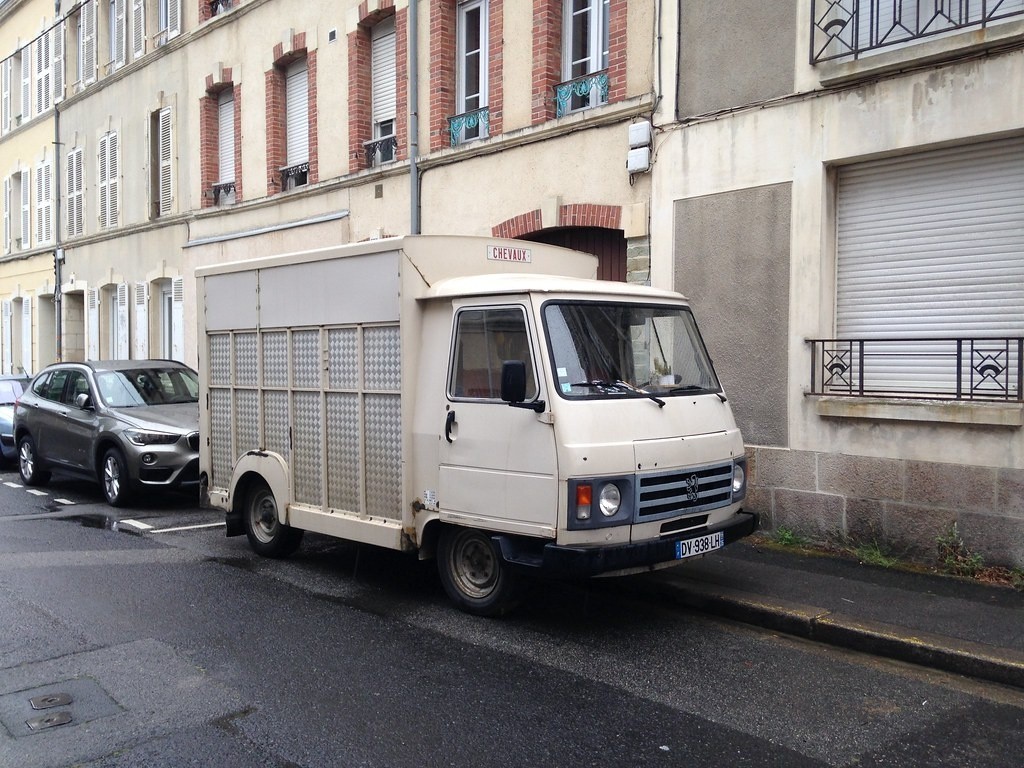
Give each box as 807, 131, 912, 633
12, 357, 199, 505
0, 373, 37, 463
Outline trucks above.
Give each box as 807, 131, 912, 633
194, 233, 758, 618
